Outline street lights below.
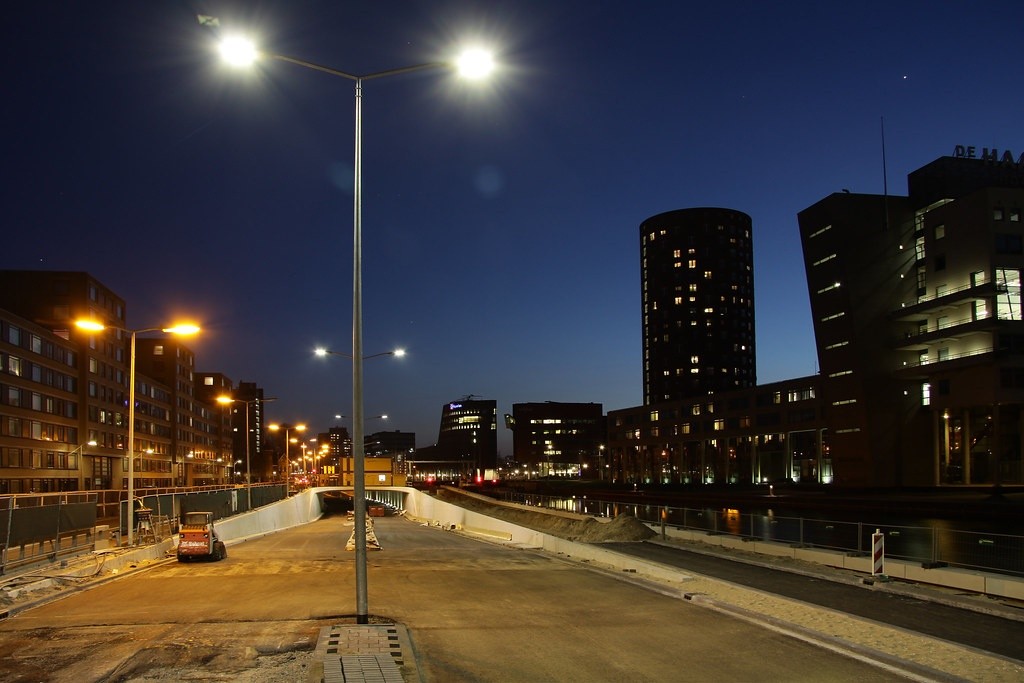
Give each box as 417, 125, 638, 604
79, 440, 97, 489
74, 315, 203, 549
289, 437, 317, 478
233, 460, 242, 484
269, 424, 307, 497
208, 27, 501, 623
182, 455, 193, 486
214, 396, 278, 512
139, 450, 154, 488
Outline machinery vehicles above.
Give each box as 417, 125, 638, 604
176, 511, 228, 561
